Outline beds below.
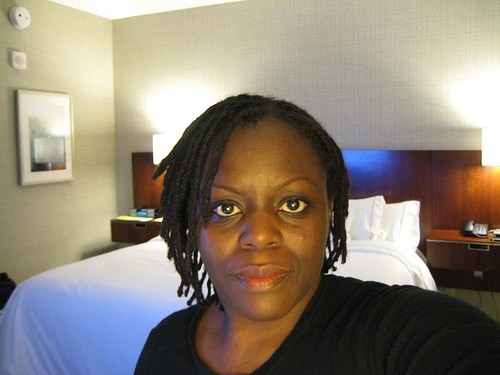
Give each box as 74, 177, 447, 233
0, 149, 438, 375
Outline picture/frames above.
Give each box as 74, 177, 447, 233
13, 89, 74, 186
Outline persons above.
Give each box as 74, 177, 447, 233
134, 94, 500, 375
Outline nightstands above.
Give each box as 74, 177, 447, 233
425, 229, 500, 273
110, 208, 163, 244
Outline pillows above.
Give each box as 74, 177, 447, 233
383, 201, 422, 249
343, 195, 386, 243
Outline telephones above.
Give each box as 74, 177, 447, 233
461, 219, 488, 238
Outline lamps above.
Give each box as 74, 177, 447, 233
482, 126, 500, 166
153, 134, 174, 165
